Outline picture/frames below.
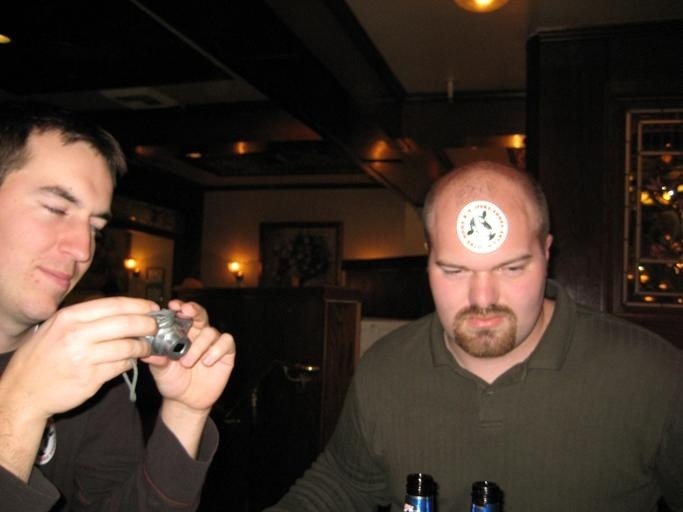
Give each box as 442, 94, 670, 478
257, 220, 344, 286
602, 74, 683, 328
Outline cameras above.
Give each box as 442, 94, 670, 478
143, 310, 193, 360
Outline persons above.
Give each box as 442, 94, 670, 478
0, 103, 239, 512
258, 158, 682, 512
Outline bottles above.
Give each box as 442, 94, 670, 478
407, 473, 438, 512
471, 480, 504, 512
